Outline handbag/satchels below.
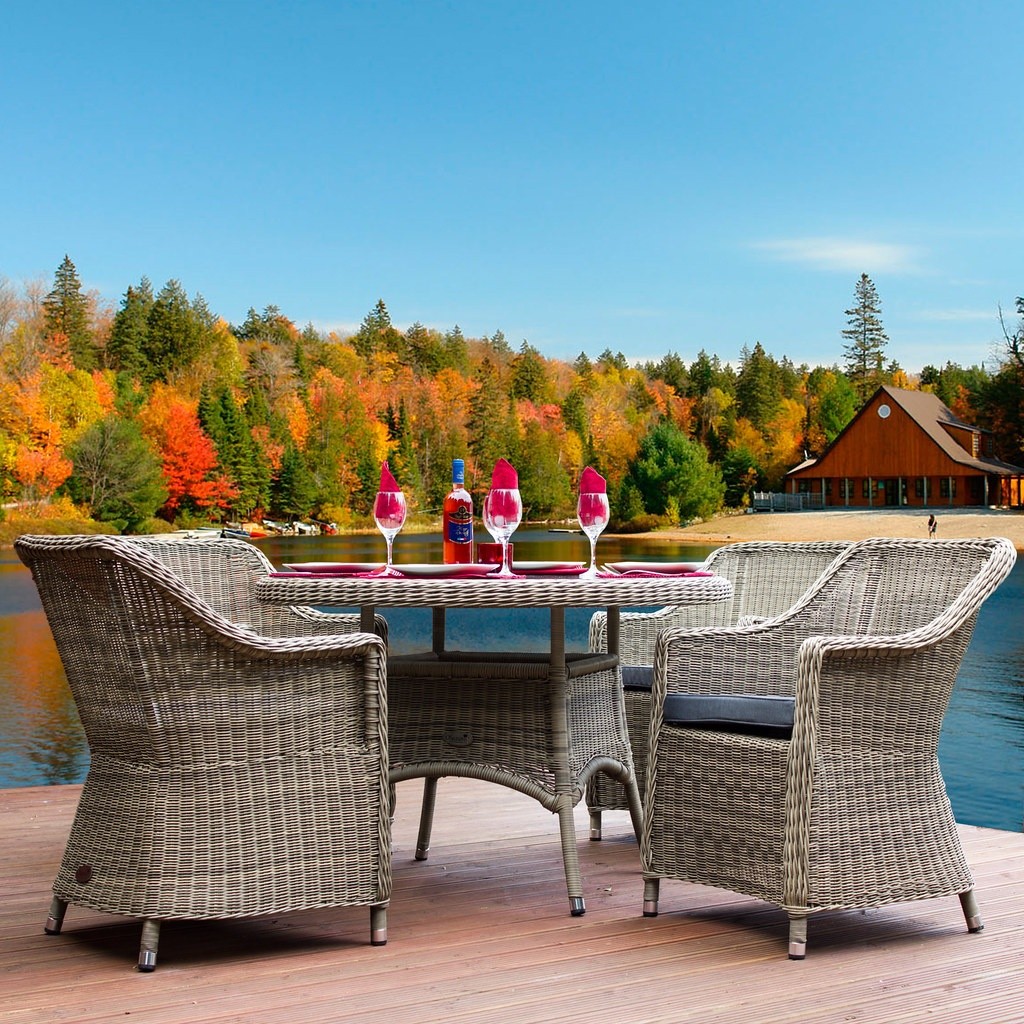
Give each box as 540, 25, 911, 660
928, 526, 933, 532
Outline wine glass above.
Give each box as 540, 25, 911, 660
483, 489, 524, 577
576, 493, 610, 579
373, 491, 407, 577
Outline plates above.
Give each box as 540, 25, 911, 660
386, 562, 501, 576
604, 560, 711, 574
282, 561, 387, 573
511, 559, 588, 568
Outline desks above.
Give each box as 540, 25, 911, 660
254, 575, 735, 917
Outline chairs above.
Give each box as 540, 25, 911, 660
642, 536, 1018, 961
588, 540, 878, 842
14, 533, 393, 973
121, 535, 389, 653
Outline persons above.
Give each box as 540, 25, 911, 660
928, 514, 937, 539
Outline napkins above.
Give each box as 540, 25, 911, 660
373, 460, 405, 526
576, 466, 606, 526
484, 457, 518, 522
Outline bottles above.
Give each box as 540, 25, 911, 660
441, 459, 472, 564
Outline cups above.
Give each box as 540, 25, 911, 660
475, 542, 513, 572
